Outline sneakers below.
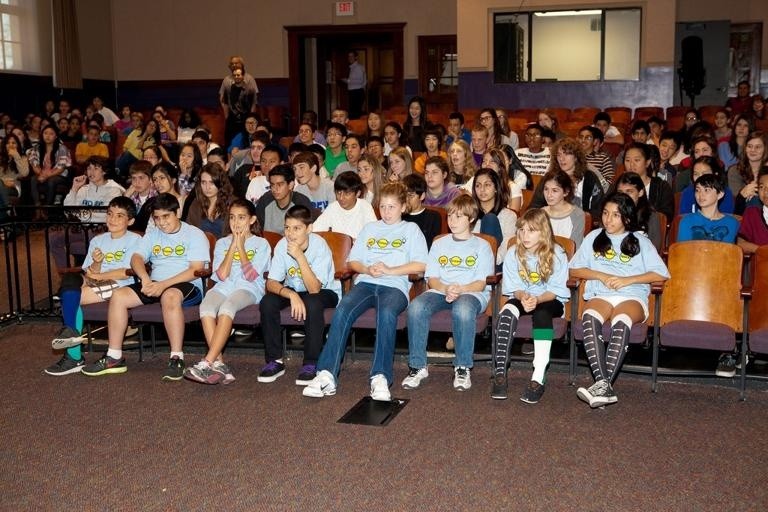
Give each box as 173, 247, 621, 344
257, 360, 285, 383
401, 368, 429, 390
162, 357, 234, 385
715, 349, 749, 378
492, 377, 508, 399
521, 339, 534, 355
295, 365, 337, 398
520, 378, 545, 404
234, 329, 253, 336
45, 327, 127, 375
370, 373, 391, 402
453, 366, 472, 392
290, 329, 306, 338
576, 380, 618, 409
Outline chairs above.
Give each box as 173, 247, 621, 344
0, 93, 767, 392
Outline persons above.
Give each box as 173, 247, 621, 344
185, 199, 268, 388
81, 193, 206, 382
2, 96, 175, 242
219, 56, 258, 112
512, 110, 681, 256
677, 81, 766, 252
42, 197, 142, 376
568, 192, 666, 408
302, 183, 428, 403
222, 68, 257, 139
402, 195, 494, 392
175, 109, 365, 239
490, 208, 568, 405
257, 205, 339, 387
715, 166, 768, 379
358, 98, 534, 271
342, 50, 368, 118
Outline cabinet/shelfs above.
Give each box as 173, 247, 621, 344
495, 23, 524, 82
